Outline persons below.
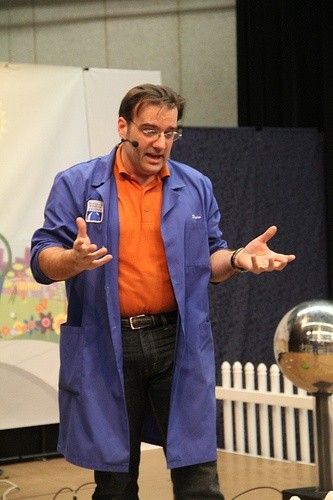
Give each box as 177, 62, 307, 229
30, 83, 298, 500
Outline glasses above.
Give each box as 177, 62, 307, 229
130, 117, 183, 141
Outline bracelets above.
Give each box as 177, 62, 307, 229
230, 247, 247, 272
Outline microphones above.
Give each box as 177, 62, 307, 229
124, 139, 139, 147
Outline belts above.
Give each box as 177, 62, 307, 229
119, 309, 180, 331
